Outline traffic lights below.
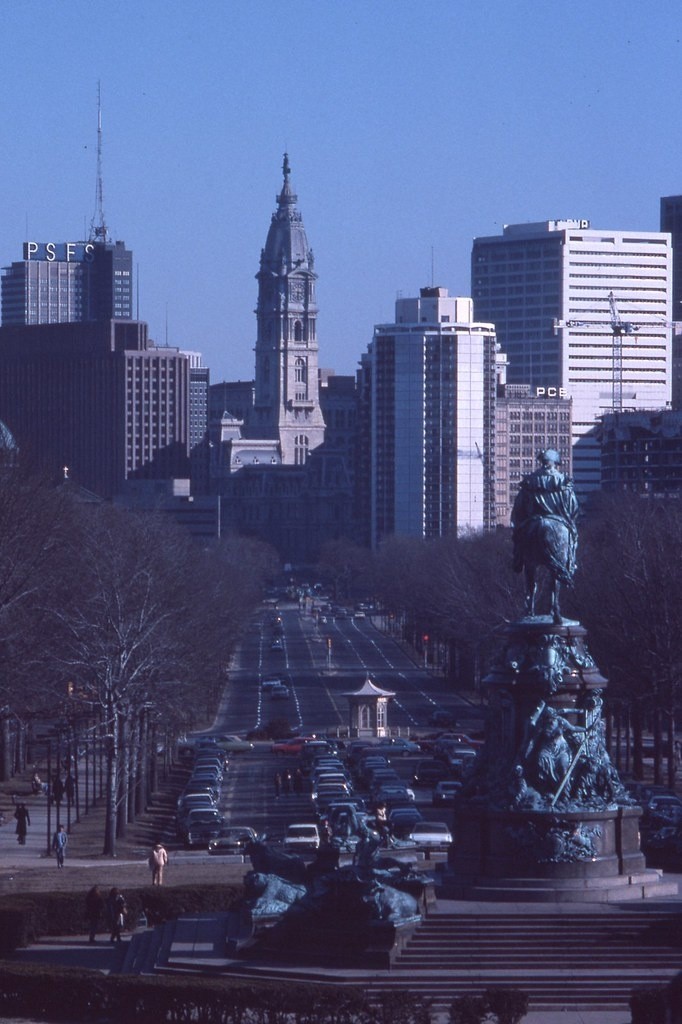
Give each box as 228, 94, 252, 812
423, 634, 430, 650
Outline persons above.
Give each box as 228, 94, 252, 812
376, 802, 394, 848
53, 825, 68, 868
34, 772, 75, 805
14, 803, 30, 845
274, 768, 302, 799
107, 888, 127, 942
319, 819, 332, 843
86, 887, 106, 941
332, 805, 383, 869
498, 700, 637, 810
510, 450, 579, 624
150, 844, 168, 886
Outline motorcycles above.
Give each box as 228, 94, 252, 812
31, 764, 48, 796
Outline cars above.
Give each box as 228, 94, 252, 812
319, 600, 368, 624
623, 782, 682, 827
262, 676, 290, 700
173, 734, 487, 860
247, 617, 283, 651
